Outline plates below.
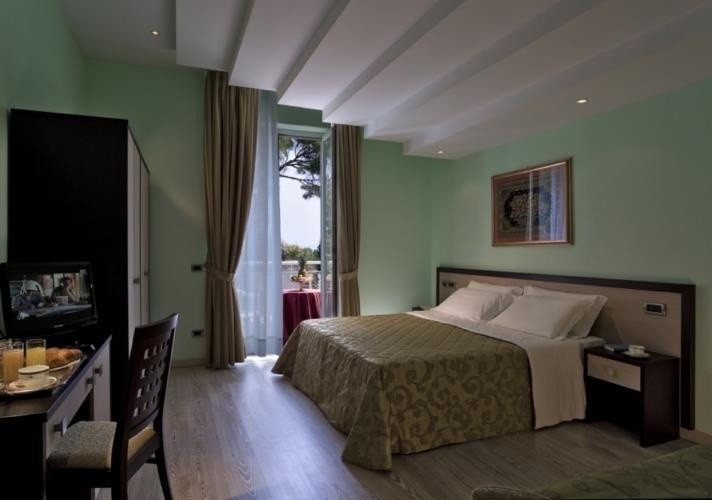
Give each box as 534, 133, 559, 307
8, 376, 57, 391
625, 351, 650, 356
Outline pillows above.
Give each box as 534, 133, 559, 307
523, 285, 607, 338
488, 294, 591, 342
434, 286, 512, 323
467, 280, 523, 296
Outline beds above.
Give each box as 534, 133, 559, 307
269, 308, 605, 473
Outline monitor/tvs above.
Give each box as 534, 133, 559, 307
0, 259, 99, 339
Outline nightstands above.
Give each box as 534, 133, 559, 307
583, 345, 681, 449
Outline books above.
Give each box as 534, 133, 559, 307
604, 344, 628, 353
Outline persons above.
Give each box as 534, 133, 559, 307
50, 277, 80, 304
10, 276, 48, 311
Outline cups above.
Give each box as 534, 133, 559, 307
19, 364, 50, 388
2, 343, 24, 384
27, 339, 45, 367
629, 345, 644, 355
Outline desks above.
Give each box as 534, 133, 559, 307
1, 330, 112, 500
283, 288, 321, 343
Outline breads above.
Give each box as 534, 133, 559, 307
46, 347, 82, 368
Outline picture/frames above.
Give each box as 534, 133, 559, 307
491, 157, 573, 247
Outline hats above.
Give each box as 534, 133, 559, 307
21, 280, 38, 291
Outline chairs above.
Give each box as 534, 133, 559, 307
47, 312, 178, 500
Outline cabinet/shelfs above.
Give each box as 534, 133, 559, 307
8, 107, 151, 359
